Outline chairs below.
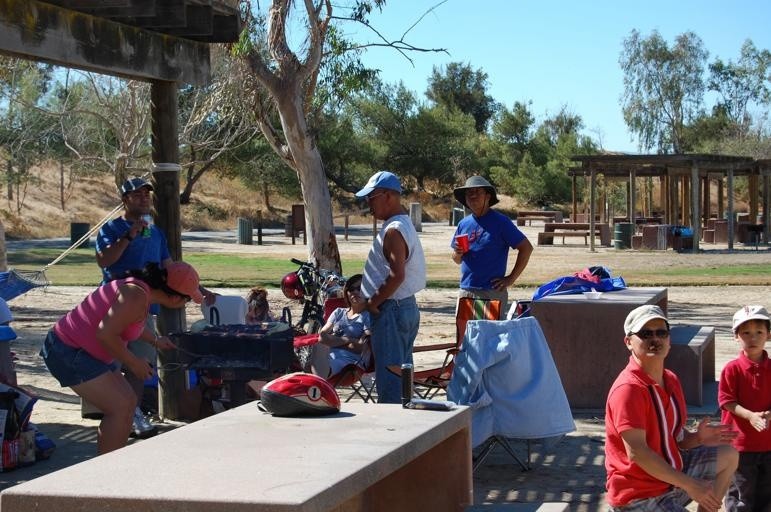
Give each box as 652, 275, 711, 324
305, 296, 374, 406
452, 312, 541, 477
386, 296, 504, 399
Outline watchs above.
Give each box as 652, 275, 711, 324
120, 230, 133, 242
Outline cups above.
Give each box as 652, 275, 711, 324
455, 233, 470, 255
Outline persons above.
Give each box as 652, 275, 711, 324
311, 274, 372, 381
354, 171, 426, 404
450, 176, 533, 320
40, 262, 204, 456
95, 178, 175, 435
717, 305, 771, 512
246, 288, 269, 323
605, 305, 740, 512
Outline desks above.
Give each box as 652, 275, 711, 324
0, 397, 475, 512
526, 287, 670, 411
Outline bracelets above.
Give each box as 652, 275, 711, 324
152, 334, 158, 347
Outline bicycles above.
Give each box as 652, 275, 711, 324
284, 255, 348, 378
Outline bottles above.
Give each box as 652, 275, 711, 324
401, 362, 413, 408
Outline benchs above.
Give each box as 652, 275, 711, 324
515, 207, 763, 252
657, 321, 716, 407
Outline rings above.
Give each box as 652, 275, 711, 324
500, 283, 504, 286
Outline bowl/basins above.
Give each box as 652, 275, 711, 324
583, 292, 603, 299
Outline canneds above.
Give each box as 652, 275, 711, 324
139, 214, 153, 239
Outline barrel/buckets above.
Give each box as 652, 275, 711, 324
614, 223, 635, 249
284, 216, 299, 238
71, 222, 91, 249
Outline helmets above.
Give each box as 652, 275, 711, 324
280, 271, 305, 300
260, 372, 341, 417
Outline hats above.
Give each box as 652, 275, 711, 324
161, 261, 204, 304
121, 177, 154, 197
355, 170, 402, 198
732, 304, 771, 334
453, 175, 500, 211
623, 304, 670, 337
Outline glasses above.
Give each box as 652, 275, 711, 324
626, 328, 669, 340
